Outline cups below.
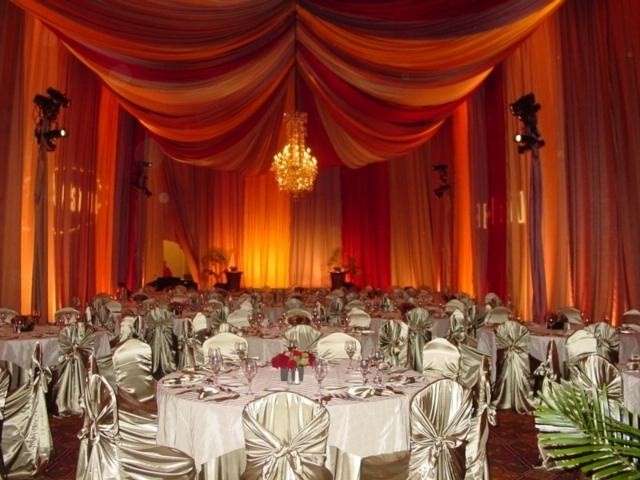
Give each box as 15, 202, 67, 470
107, 281, 232, 317
360, 293, 448, 319
618, 329, 639, 366
513, 309, 611, 333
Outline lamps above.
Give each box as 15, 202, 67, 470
509, 92, 546, 163
128, 159, 155, 199
428, 163, 452, 200
34, 84, 72, 152
270, 112, 319, 204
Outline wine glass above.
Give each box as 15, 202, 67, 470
2, 308, 80, 340
244, 309, 352, 335
208, 339, 383, 396
